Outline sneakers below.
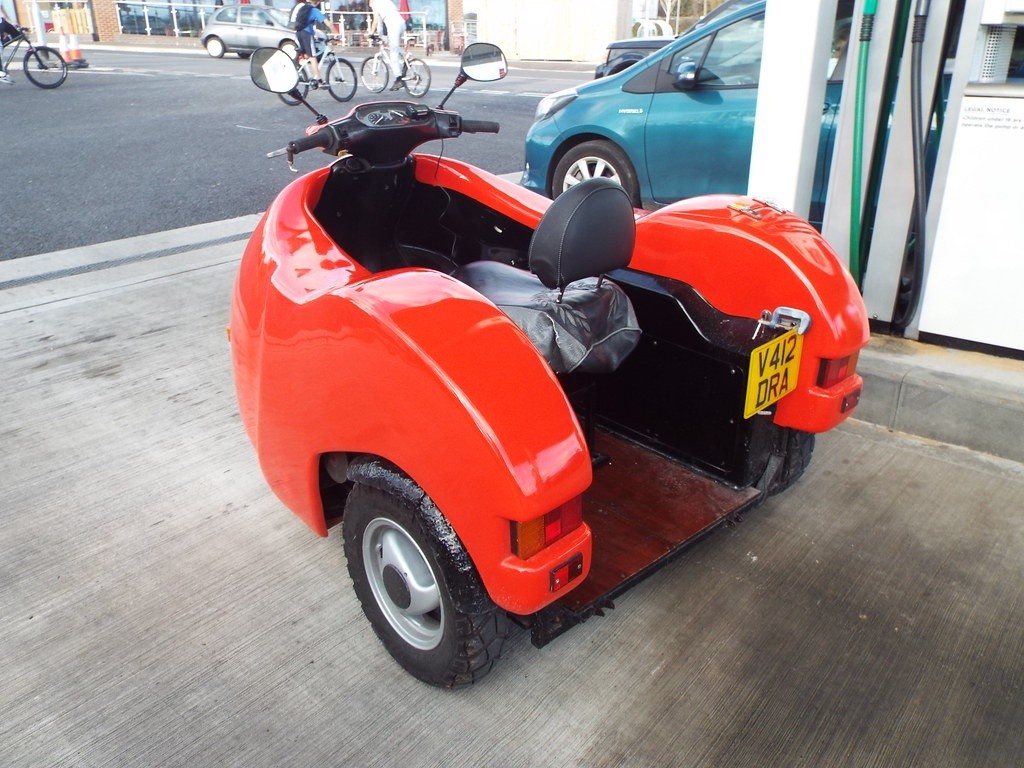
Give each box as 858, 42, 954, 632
391, 80, 405, 91
0, 71, 16, 84
309, 78, 331, 91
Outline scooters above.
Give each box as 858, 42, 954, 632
229, 43, 870, 690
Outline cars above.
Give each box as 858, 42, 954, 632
120, 15, 172, 35
517, 0, 969, 214
201, 3, 327, 58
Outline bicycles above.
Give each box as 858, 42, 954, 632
0, 28, 68, 88
276, 39, 358, 106
361, 33, 433, 99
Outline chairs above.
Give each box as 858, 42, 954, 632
454, 177, 643, 471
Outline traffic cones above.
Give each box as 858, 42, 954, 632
59, 26, 88, 68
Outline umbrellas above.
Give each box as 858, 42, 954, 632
240, 0, 250, 5
399, 0, 410, 22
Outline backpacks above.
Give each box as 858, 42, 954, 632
295, 5, 315, 30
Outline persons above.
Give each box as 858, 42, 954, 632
296, 0, 339, 90
368, 0, 406, 90
0, 0, 21, 85
